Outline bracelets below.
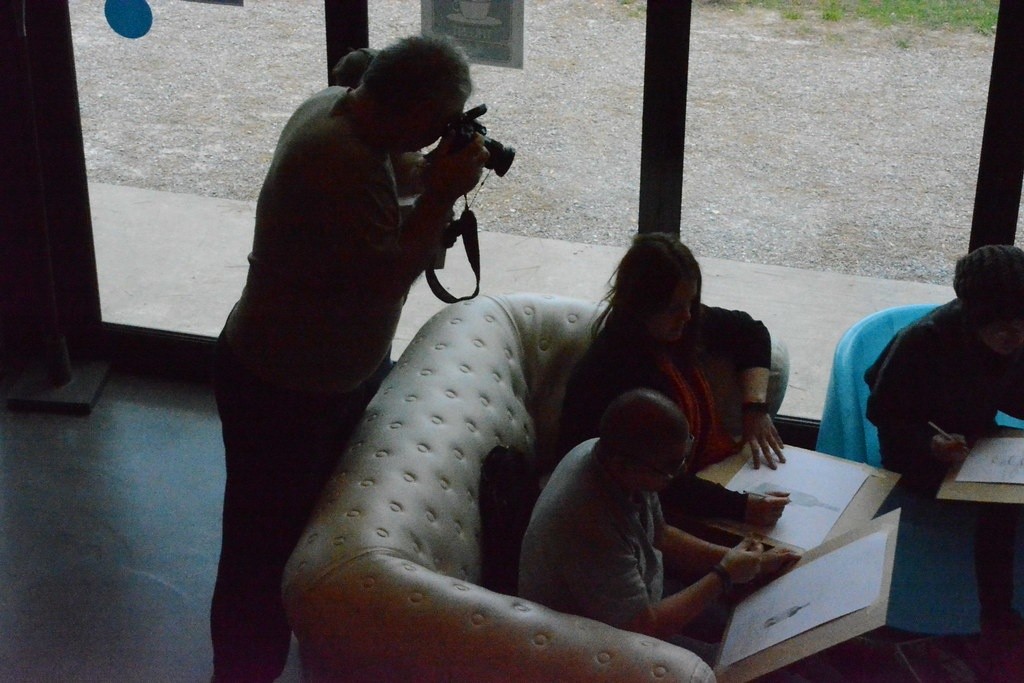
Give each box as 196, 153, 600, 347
708, 561, 735, 607
738, 401, 771, 414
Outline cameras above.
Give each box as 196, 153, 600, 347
446, 104, 515, 177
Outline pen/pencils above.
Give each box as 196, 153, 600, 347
742, 489, 793, 503
927, 419, 970, 453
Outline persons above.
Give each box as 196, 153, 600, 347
864, 246, 1024, 683
206, 43, 490, 681
555, 230, 791, 550
334, 48, 426, 427
516, 388, 800, 667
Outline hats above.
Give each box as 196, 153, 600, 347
953, 242, 1023, 322
330, 46, 379, 74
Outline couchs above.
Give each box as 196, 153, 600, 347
814, 304, 1024, 635
276, 289, 792, 683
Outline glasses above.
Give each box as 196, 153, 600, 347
611, 434, 696, 482
